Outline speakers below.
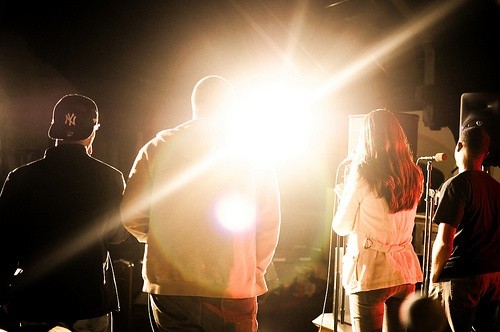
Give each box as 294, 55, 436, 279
459, 92, 500, 168
348, 112, 419, 181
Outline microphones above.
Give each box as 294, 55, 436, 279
420, 152, 447, 162
342, 158, 351, 166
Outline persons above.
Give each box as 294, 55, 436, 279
430, 126, 500, 332
332, 108, 423, 332
120, 76, 281, 332
0, 95, 129, 332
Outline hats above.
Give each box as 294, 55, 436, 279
48, 93, 99, 139
